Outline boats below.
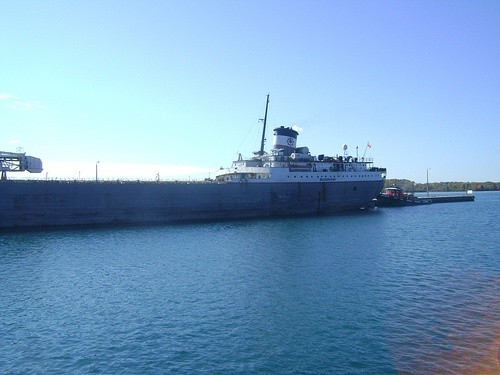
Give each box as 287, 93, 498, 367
376, 184, 431, 204
289, 164, 312, 171
0, 94, 386, 228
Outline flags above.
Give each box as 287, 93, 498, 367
368, 143, 371, 148
